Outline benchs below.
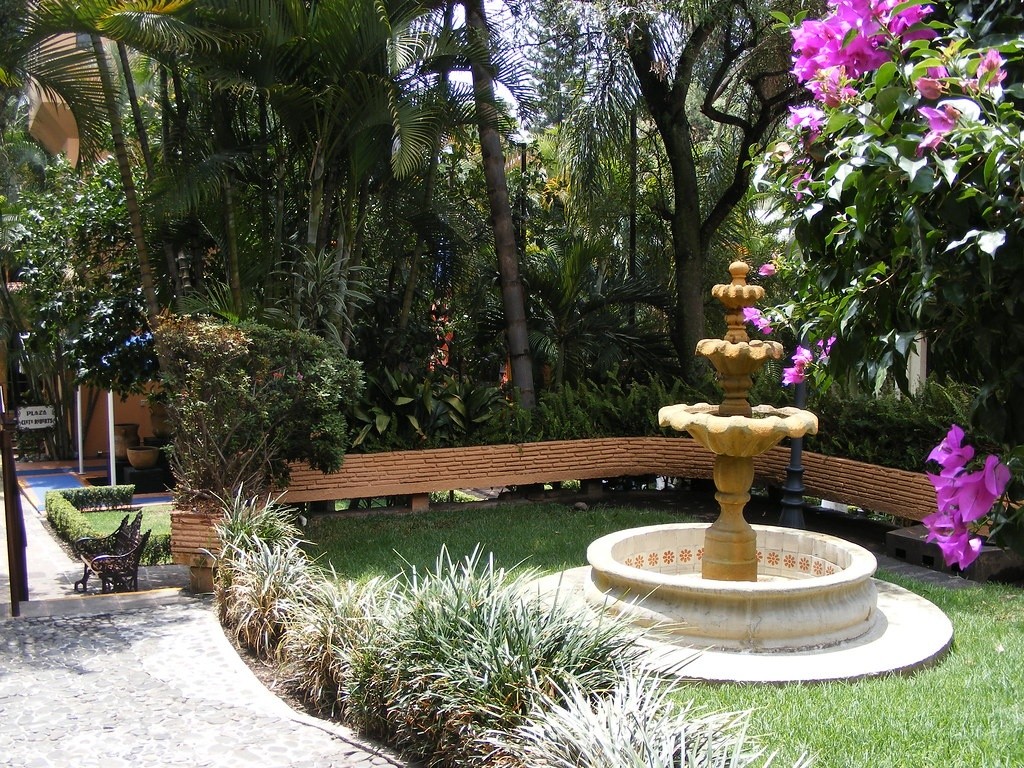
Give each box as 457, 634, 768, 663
74, 510, 151, 595
